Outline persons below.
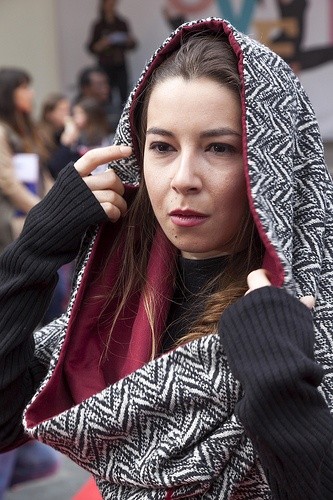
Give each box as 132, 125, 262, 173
0, 66, 52, 248
62, 99, 106, 157
78, 69, 111, 107
86, 1, 137, 109
1, 17, 333, 499
36, 96, 68, 144
